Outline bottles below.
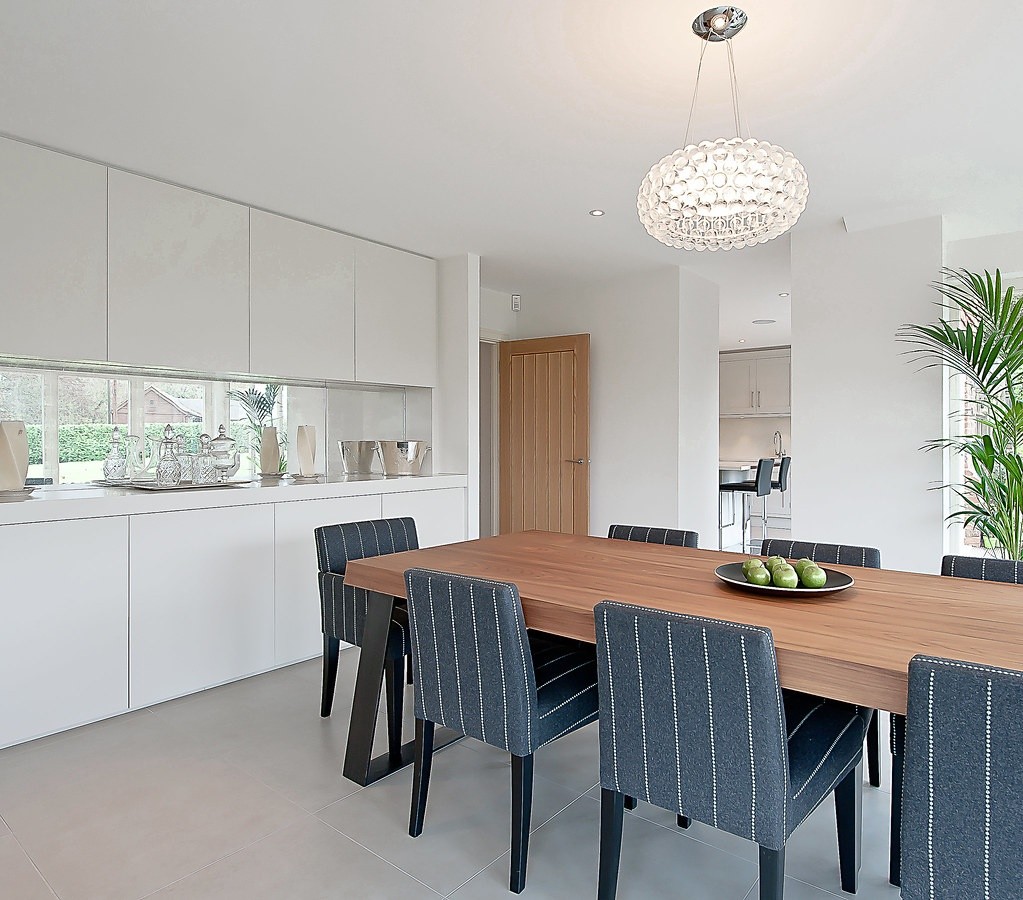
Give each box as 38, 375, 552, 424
103, 426, 146, 486
191, 423, 237, 488
156, 424, 181, 488
176, 435, 193, 483
130, 434, 162, 484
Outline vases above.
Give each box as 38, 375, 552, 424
256, 426, 289, 478
0, 421, 40, 498
290, 426, 323, 482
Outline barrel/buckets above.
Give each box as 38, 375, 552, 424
337, 439, 375, 474
375, 440, 432, 476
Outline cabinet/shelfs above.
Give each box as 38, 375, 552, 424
0, 136, 466, 750
719, 348, 790, 418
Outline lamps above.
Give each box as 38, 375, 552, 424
638, 8, 808, 251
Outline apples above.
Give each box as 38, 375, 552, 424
742, 555, 827, 588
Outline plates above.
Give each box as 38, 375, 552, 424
290, 473, 324, 481
0, 487, 42, 497
714, 561, 856, 598
257, 472, 289, 478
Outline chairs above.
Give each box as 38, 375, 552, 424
899, 652, 1023, 900
940, 555, 1023, 585
401, 568, 638, 894
314, 517, 421, 716
595, 601, 823, 900
758, 539, 882, 788
607, 524, 699, 548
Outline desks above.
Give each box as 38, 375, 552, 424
341, 528, 1023, 884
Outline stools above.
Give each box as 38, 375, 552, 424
718, 458, 774, 530
748, 457, 791, 523
